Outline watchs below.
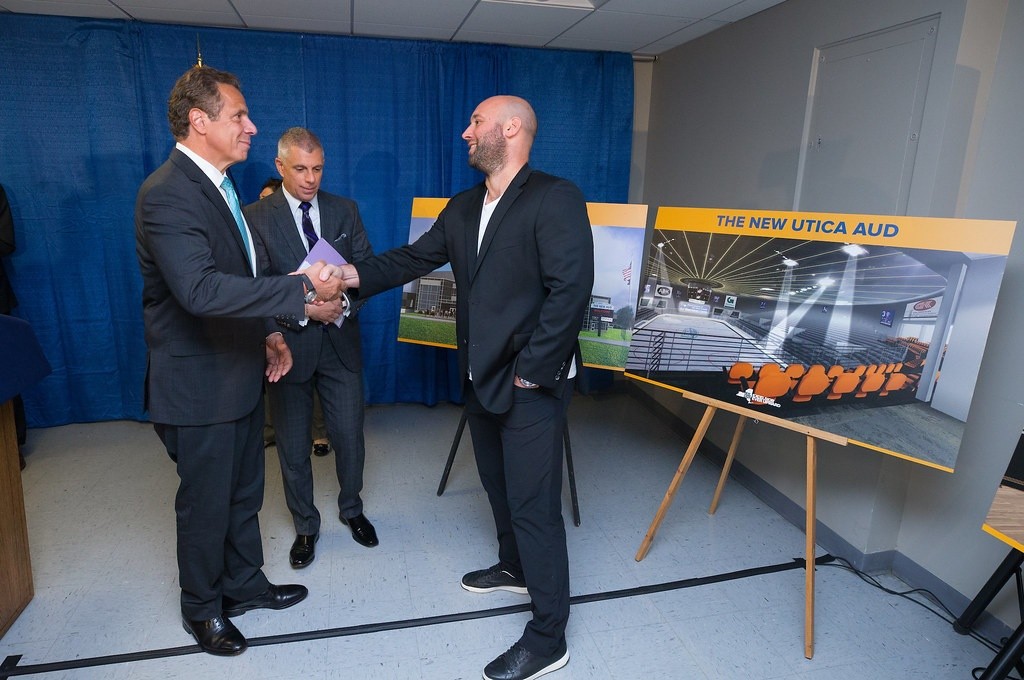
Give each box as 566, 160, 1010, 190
300, 273, 318, 304
518, 374, 539, 388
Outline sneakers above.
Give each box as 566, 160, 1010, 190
461, 562, 529, 594
483, 641, 570, 680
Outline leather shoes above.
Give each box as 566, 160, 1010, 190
290, 531, 319, 568
182, 613, 248, 656
339, 512, 379, 547
223, 584, 308, 617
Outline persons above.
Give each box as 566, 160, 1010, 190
0, 184, 26, 471
136, 65, 348, 658
288, 96, 595, 680
243, 126, 378, 568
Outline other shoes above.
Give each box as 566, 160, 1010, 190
313, 438, 331, 456
263, 425, 276, 446
19, 449, 26, 471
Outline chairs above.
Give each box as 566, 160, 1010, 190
722, 335, 950, 414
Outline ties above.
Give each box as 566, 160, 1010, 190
298, 201, 319, 252
221, 177, 252, 267
